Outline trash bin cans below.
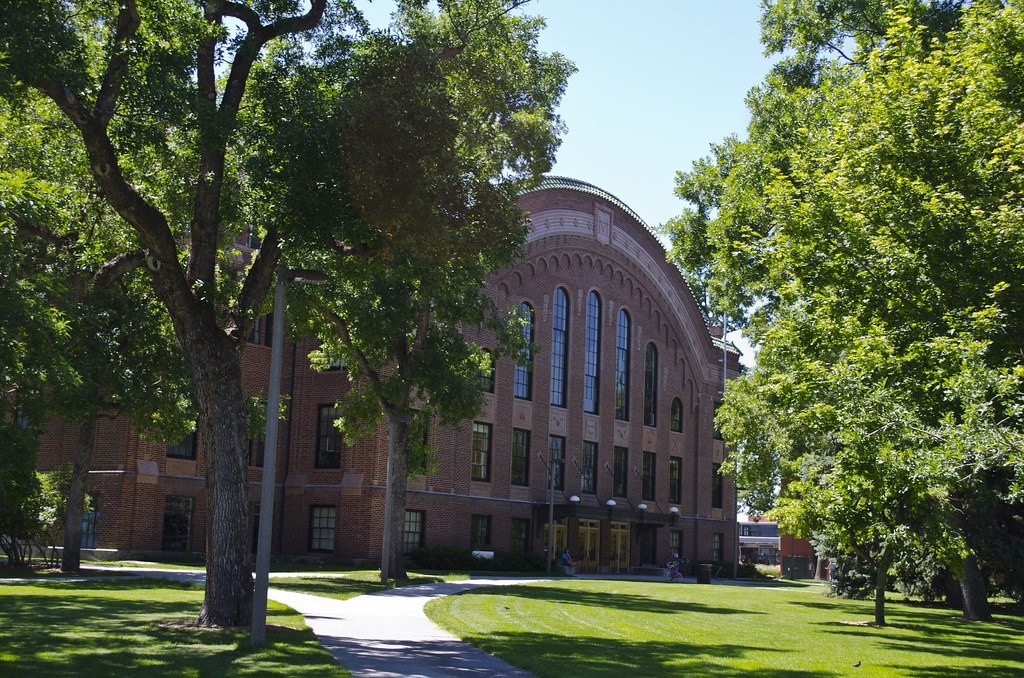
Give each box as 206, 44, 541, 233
697, 564, 712, 584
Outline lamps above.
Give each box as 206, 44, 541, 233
670, 507, 678, 513
607, 500, 617, 505
638, 503, 647, 509
570, 495, 580, 502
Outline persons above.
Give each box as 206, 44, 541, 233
669, 552, 693, 581
561, 547, 579, 566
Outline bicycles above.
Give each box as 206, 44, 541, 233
664, 563, 682, 582
